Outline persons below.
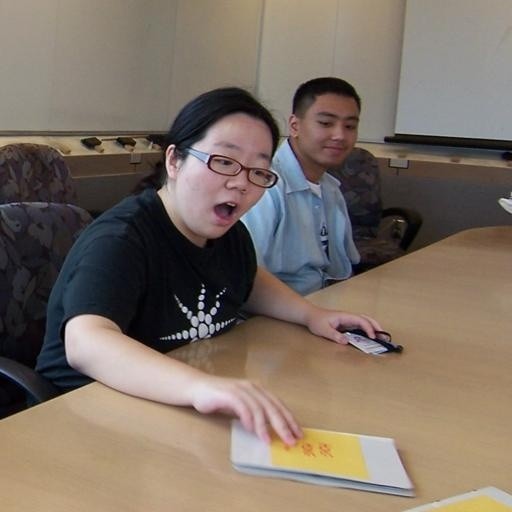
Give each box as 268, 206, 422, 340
239, 78, 363, 302
32, 85, 388, 448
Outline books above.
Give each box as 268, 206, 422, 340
228, 417, 415, 499
409, 485, 511, 512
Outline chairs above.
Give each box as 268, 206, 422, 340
330, 150, 421, 268
2, 202, 112, 406
3, 144, 77, 203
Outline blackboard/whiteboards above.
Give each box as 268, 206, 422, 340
395, 1, 512, 148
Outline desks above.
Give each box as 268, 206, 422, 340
0, 226, 512, 512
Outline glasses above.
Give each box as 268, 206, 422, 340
181, 145, 278, 189
